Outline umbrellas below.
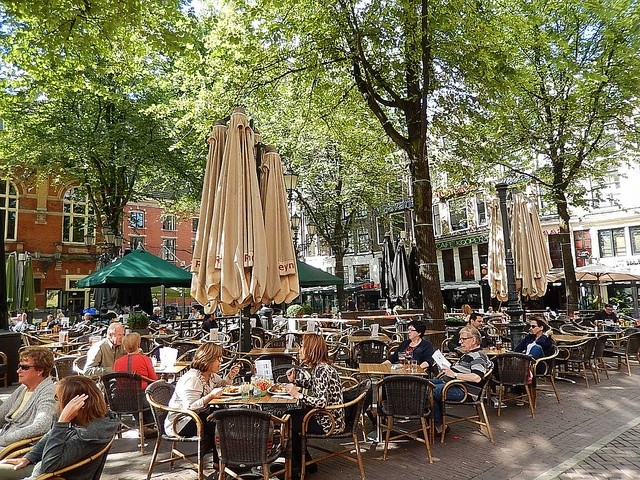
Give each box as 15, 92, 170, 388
487, 194, 553, 302
190, 107, 299, 352
378, 227, 397, 312
391, 231, 411, 311
559, 261, 640, 308
20, 252, 36, 322
3, 246, 18, 314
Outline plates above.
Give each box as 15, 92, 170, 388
223, 386, 253, 395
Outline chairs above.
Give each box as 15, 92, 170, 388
440, 361, 495, 443
497, 352, 536, 418
486, 328, 502, 343
601, 331, 640, 377
54, 355, 78, 381
281, 333, 302, 347
154, 329, 175, 343
326, 326, 359, 342
19, 331, 51, 346
199, 332, 231, 348
206, 408, 292, 480
218, 336, 245, 374
325, 333, 351, 369
572, 318, 596, 330
559, 323, 585, 337
144, 378, 213, 480
330, 363, 371, 390
553, 338, 598, 389
355, 339, 388, 368
145, 344, 163, 361
565, 334, 610, 383
387, 345, 399, 360
263, 336, 286, 348
0, 435, 43, 459
251, 326, 271, 349
3, 433, 116, 480
620, 328, 640, 337
529, 345, 560, 408
102, 371, 155, 454
378, 324, 405, 341
441, 337, 453, 353
376, 374, 437, 464
547, 319, 567, 334
0, 352, 8, 387
487, 317, 505, 333
221, 348, 255, 375
73, 355, 87, 376
298, 378, 371, 480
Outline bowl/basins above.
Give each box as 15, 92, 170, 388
267, 386, 301, 395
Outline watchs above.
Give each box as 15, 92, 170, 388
297, 392, 306, 400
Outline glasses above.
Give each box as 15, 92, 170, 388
18, 364, 36, 370
529, 325, 538, 328
459, 337, 473, 343
407, 328, 416, 332
217, 356, 223, 361
54, 393, 59, 402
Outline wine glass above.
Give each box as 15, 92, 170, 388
406, 351, 413, 371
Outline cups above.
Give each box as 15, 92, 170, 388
496, 341, 502, 349
412, 360, 417, 369
254, 387, 261, 399
242, 382, 249, 400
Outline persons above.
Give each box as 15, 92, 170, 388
591, 302, 620, 368
46, 314, 57, 327
113, 332, 159, 439
164, 342, 240, 469
75, 313, 91, 330
386, 321, 433, 430
10, 313, 27, 332
427, 326, 490, 438
0, 374, 108, 480
458, 302, 473, 320
286, 334, 331, 474
516, 318, 554, 407
188, 305, 200, 319
85, 324, 125, 376
448, 313, 496, 358
1, 345, 59, 450
150, 308, 160, 321
203, 313, 217, 330
